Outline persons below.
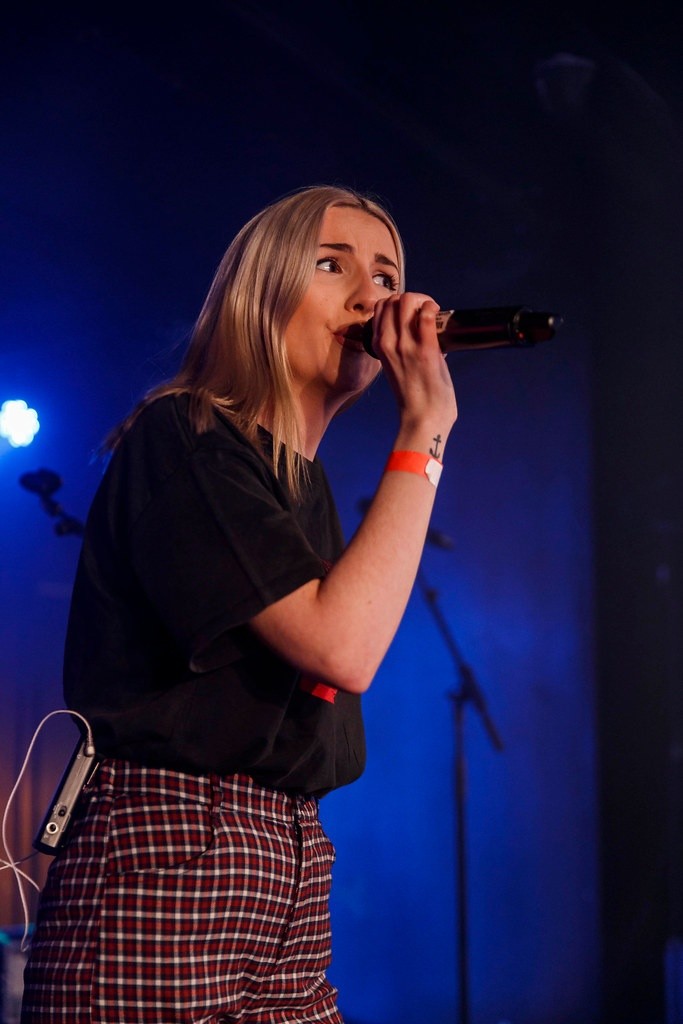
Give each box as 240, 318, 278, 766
17, 181, 461, 1023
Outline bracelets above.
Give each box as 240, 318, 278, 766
384, 449, 445, 488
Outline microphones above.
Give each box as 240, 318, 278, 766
358, 499, 455, 548
362, 306, 565, 360
